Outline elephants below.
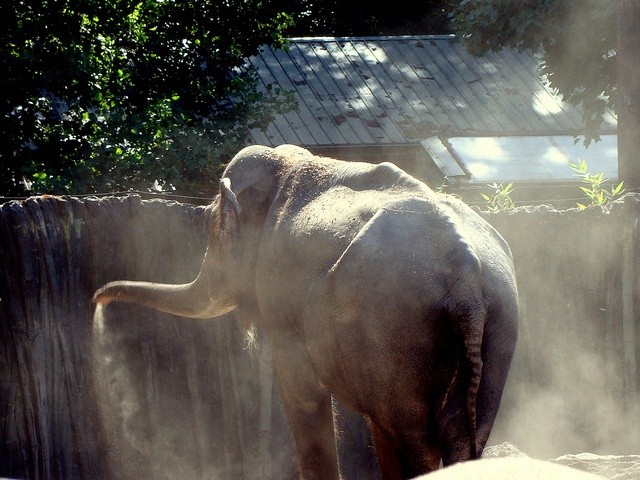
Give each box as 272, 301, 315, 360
90, 144, 522, 480
